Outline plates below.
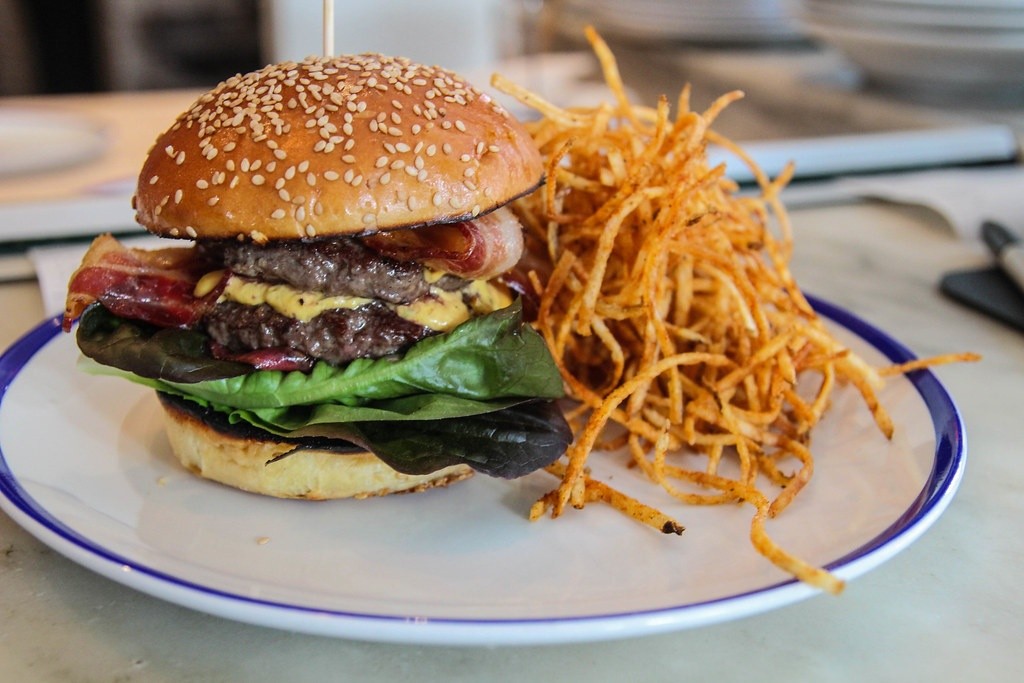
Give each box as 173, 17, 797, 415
527, 0, 1024, 89
0, 288, 968, 647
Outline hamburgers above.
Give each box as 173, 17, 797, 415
61, 52, 572, 503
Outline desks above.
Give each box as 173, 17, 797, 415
0, 56, 1023, 681
